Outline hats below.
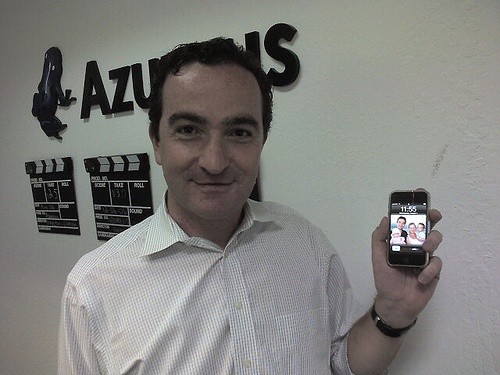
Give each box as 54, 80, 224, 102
392, 227, 400, 234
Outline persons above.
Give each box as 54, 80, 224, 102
391, 216, 409, 244
390, 228, 407, 245
406, 222, 423, 244
415, 222, 425, 242
54, 35, 447, 374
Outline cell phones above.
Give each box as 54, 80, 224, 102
387, 188, 431, 269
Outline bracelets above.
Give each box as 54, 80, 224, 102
371, 302, 417, 337
